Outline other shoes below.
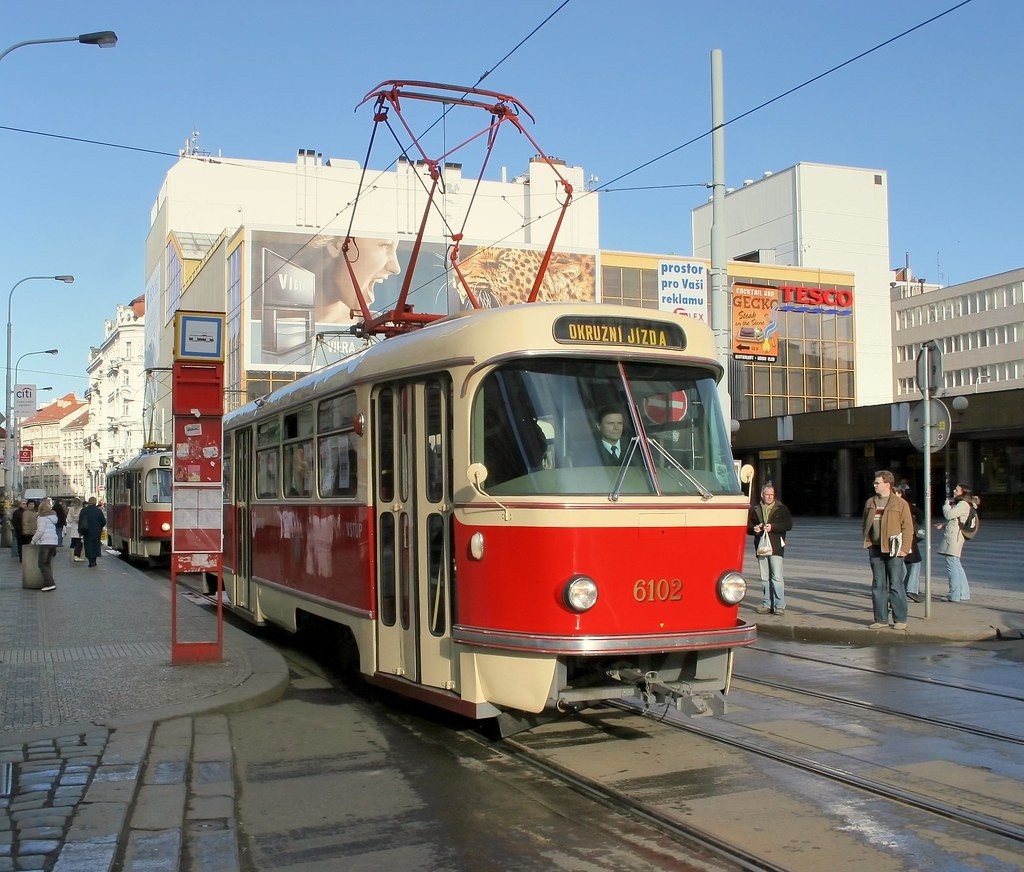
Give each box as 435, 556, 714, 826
774, 608, 785, 615
759, 606, 773, 614
907, 592, 922, 603
869, 622, 889, 629
88, 563, 96, 567
40, 585, 56, 591
941, 596, 952, 602
75, 556, 84, 562
894, 622, 906, 630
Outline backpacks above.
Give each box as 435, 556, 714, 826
955, 499, 979, 539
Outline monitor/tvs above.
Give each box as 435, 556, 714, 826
261, 248, 316, 308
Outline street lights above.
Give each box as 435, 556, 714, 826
1, 275, 76, 548
14, 349, 58, 500
19, 387, 53, 500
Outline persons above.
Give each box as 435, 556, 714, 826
936, 482, 981, 602
495, 408, 547, 480
51, 498, 67, 547
22, 499, 37, 545
67, 498, 88, 562
748, 485, 793, 614
97, 500, 104, 507
862, 471, 914, 630
78, 497, 107, 567
898, 480, 913, 503
893, 487, 923, 603
572, 406, 647, 466
31, 502, 58, 591
12, 499, 27, 562
308, 235, 401, 322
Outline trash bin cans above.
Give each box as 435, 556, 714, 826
22, 545, 45, 589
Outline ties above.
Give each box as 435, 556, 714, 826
872, 482, 887, 485
611, 446, 619, 459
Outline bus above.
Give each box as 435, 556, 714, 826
199, 78, 758, 740
105, 366, 175, 569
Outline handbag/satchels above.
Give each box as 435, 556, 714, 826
757, 529, 773, 556
912, 520, 925, 543
78, 508, 88, 534
